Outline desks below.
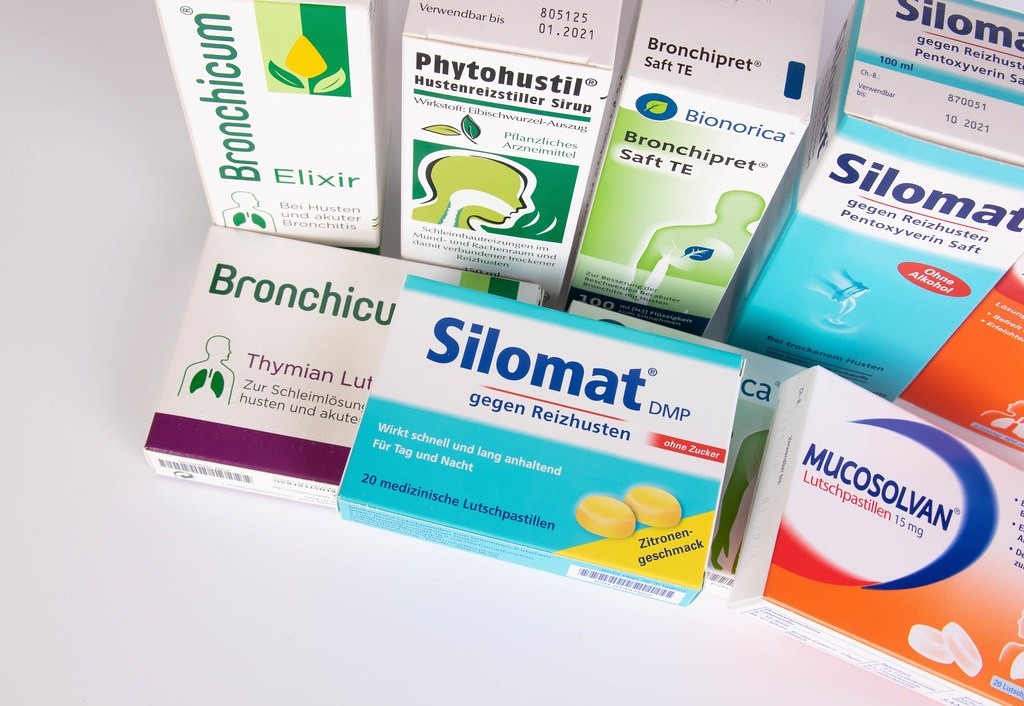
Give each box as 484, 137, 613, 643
1, 1, 1024, 706
